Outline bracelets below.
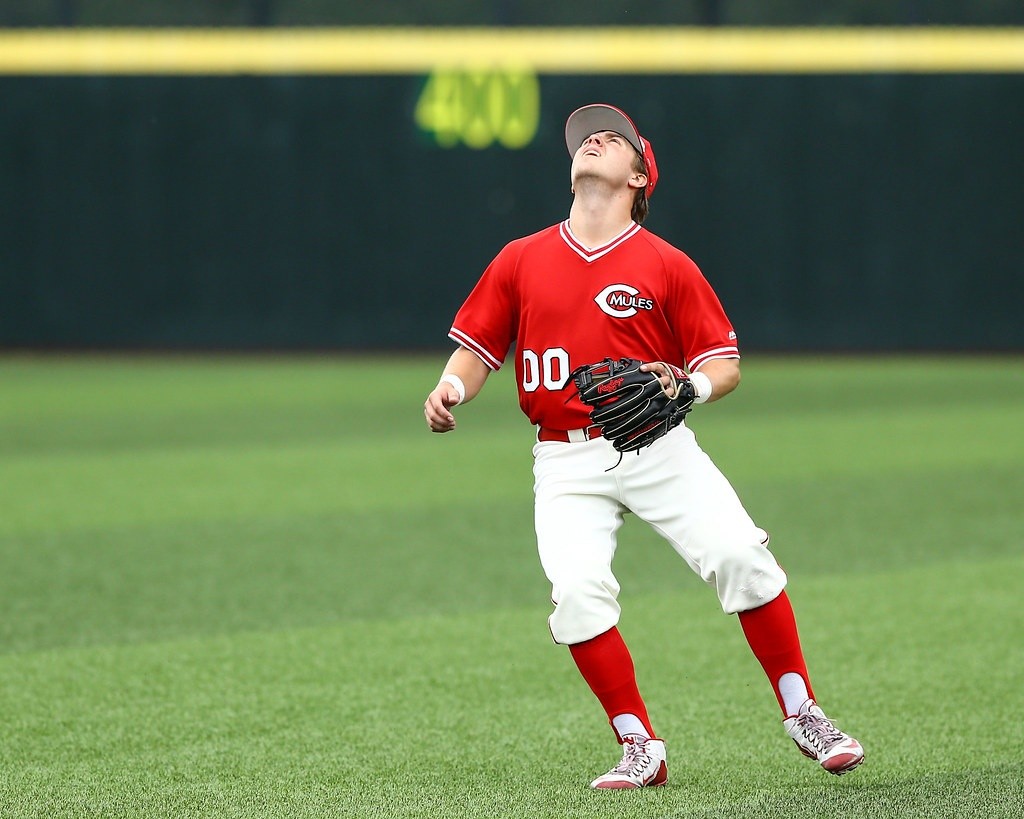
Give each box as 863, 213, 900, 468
687, 372, 711, 404
439, 374, 465, 406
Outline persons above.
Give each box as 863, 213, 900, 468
424, 103, 866, 790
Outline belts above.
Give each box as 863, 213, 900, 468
538, 425, 607, 443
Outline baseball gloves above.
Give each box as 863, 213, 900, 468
561, 356, 702, 478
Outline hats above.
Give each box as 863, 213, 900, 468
565, 103, 659, 199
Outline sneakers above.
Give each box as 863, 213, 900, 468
590, 735, 667, 790
783, 699, 863, 777
